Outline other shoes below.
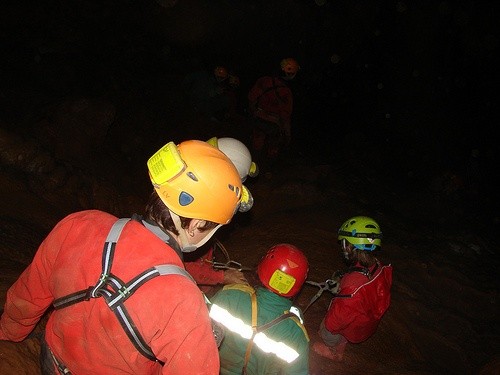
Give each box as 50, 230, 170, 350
313, 342, 346, 361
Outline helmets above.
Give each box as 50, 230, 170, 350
280, 58, 299, 74
147, 139, 242, 225
206, 137, 258, 184
336, 216, 382, 252
257, 244, 309, 298
214, 66, 229, 79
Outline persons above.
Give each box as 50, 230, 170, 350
313, 216, 393, 361
207, 243, 310, 375
184, 136, 258, 297
0, 140, 253, 375
207, 65, 240, 121
248, 57, 302, 163
160, 45, 172, 63
